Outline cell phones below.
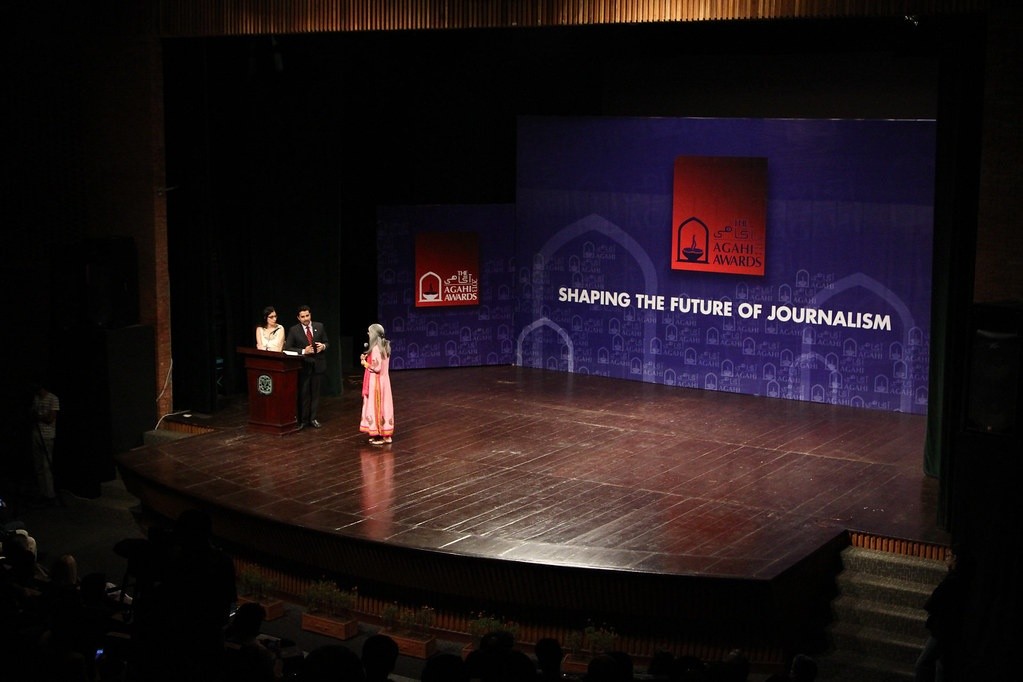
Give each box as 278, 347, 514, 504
95, 648, 103, 661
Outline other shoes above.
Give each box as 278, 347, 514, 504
368, 436, 393, 445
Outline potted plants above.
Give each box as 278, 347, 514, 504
296, 579, 359, 641
559, 623, 618, 674
235, 563, 290, 622
376, 603, 438, 660
461, 617, 520, 661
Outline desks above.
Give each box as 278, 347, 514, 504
235, 346, 305, 439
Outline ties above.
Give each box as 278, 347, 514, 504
305, 325, 313, 347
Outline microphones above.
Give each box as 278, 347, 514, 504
271, 325, 282, 335
364, 343, 368, 354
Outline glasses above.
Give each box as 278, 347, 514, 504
267, 315, 277, 319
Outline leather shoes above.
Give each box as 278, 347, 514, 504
311, 419, 322, 429
296, 422, 307, 431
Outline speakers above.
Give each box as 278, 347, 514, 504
948, 305, 1023, 548
91, 318, 157, 458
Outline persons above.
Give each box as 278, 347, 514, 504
255, 306, 286, 355
284, 305, 330, 431
1, 523, 848, 682
358, 324, 395, 446
25, 379, 64, 504
